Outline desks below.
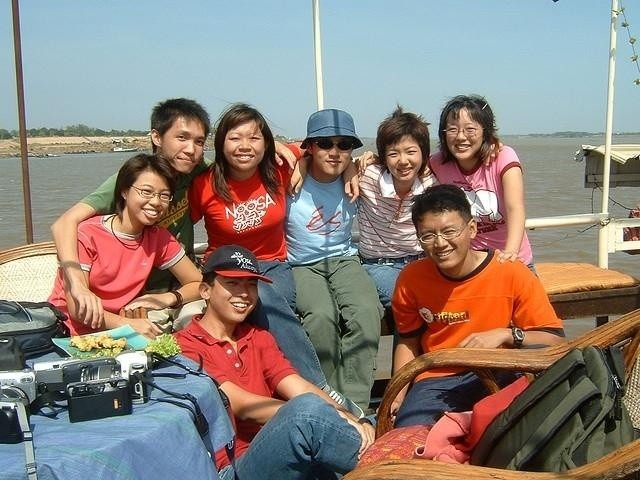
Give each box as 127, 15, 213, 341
0, 346, 236, 480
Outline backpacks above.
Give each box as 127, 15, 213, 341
0, 300, 70, 369
470, 346, 640, 473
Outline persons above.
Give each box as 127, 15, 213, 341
49, 98, 297, 335
355, 95, 538, 280
388, 183, 566, 430
283, 110, 431, 415
288, 106, 441, 332
187, 102, 364, 420
46, 152, 203, 341
168, 244, 376, 479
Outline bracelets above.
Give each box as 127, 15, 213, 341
168, 290, 183, 309
358, 418, 373, 425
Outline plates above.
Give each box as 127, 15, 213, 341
51, 325, 150, 361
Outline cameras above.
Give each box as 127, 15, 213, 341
0, 385, 30, 444
67, 376, 131, 422
32, 358, 81, 399
116, 348, 154, 404
63, 358, 121, 389
0, 368, 38, 405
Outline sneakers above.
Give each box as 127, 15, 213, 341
328, 388, 364, 419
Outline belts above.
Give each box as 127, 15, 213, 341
362, 254, 426, 264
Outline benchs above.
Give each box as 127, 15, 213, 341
370, 260, 640, 398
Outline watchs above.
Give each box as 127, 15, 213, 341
507, 325, 524, 349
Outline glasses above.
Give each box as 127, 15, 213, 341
443, 128, 483, 133
417, 223, 466, 244
131, 185, 174, 201
315, 140, 352, 150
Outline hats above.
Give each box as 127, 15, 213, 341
201, 245, 272, 283
300, 109, 363, 149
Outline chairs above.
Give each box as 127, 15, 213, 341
339, 307, 640, 480
